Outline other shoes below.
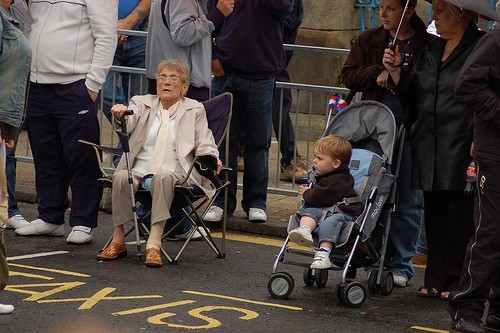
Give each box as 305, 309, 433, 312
289, 228, 314, 247
97, 241, 128, 260
416, 286, 450, 301
391, 273, 407, 287
310, 251, 331, 269
238, 157, 245, 171
280, 154, 308, 182
144, 247, 163, 267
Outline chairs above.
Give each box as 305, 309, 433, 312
77, 92, 234, 266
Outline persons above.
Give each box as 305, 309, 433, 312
289, 134, 364, 270
0, 0, 313, 314
97, 59, 222, 267
341, 0, 500, 333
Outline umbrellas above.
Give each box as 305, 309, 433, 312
388, 0, 500, 67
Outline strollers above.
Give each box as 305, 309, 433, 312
265, 101, 406, 306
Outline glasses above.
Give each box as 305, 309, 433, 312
155, 74, 183, 85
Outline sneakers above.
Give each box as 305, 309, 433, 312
14, 219, 66, 236
485, 313, 500, 332
448, 318, 488, 333
202, 205, 224, 221
7, 214, 31, 229
248, 207, 267, 222
66, 226, 94, 245
166, 224, 210, 241
